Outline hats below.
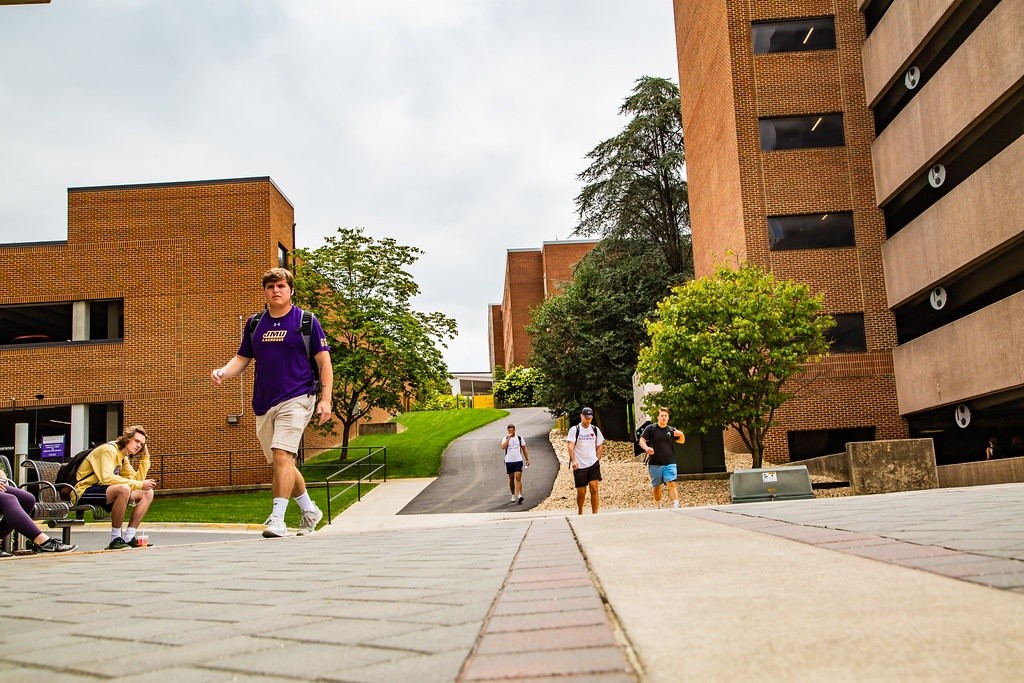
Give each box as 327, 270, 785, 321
582, 407, 593, 419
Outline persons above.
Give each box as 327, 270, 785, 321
639, 407, 685, 510
500, 424, 529, 503
566, 407, 605, 515
210, 268, 334, 537
0, 469, 78, 557
70, 425, 157, 549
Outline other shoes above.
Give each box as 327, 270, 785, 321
518, 496, 524, 505
297, 501, 323, 535
127, 537, 154, 549
109, 537, 132, 550
261, 515, 287, 538
511, 495, 516, 502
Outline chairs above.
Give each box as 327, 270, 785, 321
0, 454, 70, 557
20, 459, 113, 546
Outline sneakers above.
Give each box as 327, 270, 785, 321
32, 537, 78, 553
0, 550, 15, 559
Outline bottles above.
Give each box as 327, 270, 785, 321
137, 536, 149, 548
525, 462, 529, 468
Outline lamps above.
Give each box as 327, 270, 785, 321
227, 415, 239, 424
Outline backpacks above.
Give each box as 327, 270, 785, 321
55, 448, 95, 501
